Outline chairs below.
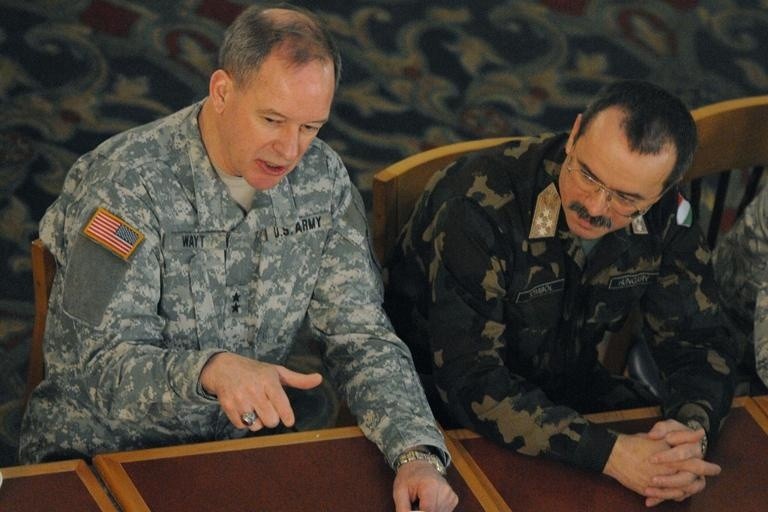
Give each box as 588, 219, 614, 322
368, 134, 533, 266
660, 95, 766, 258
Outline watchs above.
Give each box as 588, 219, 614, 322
395, 450, 450, 479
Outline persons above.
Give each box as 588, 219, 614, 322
389, 79, 741, 506
17, 3, 459, 510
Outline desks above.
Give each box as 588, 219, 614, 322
0, 394, 767, 510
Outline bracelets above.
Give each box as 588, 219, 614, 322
686, 421, 708, 459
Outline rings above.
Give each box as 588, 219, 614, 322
240, 411, 258, 426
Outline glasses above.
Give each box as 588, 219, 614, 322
562, 143, 668, 221
